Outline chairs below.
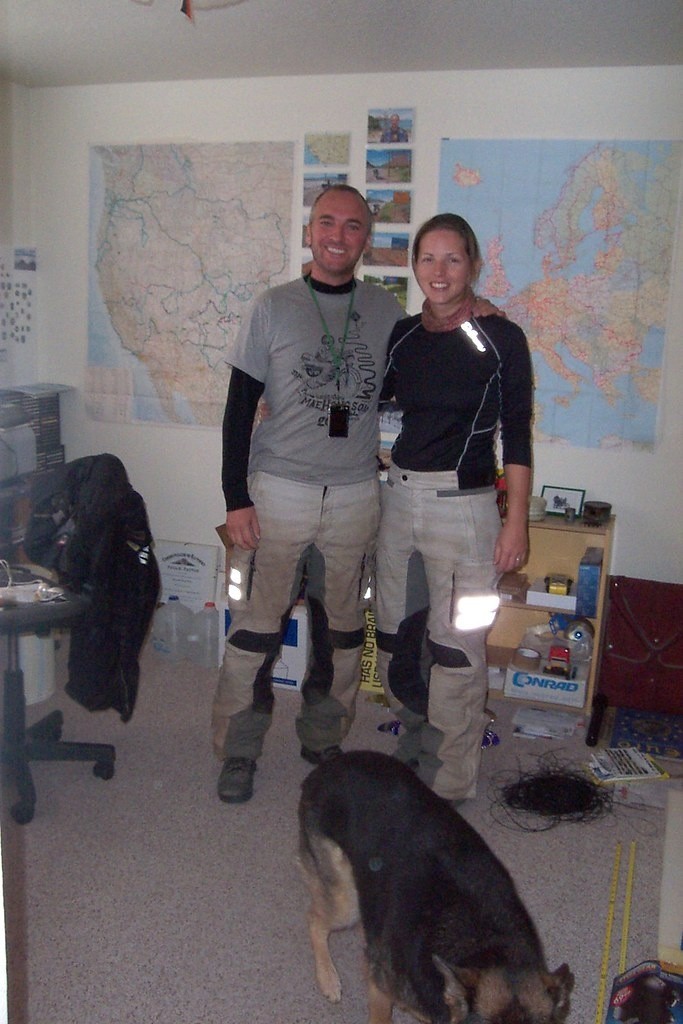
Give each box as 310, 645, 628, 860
0, 455, 127, 827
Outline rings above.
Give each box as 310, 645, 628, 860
515, 557, 522, 562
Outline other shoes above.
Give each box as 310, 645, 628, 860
300, 742, 342, 767
217, 756, 256, 803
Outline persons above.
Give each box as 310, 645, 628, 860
378, 115, 409, 142
378, 213, 533, 809
212, 183, 507, 803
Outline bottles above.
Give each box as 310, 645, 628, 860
194, 601, 219, 670
152, 594, 197, 687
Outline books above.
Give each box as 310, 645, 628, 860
10, 382, 75, 398
581, 747, 670, 785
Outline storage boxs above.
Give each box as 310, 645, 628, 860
151, 539, 219, 607
498, 572, 531, 603
605, 959, 683, 1024
213, 524, 314, 692
611, 706, 683, 759
487, 643, 513, 690
576, 546, 603, 617
504, 635, 592, 706
526, 576, 578, 611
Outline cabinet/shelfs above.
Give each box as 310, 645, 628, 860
487, 512, 616, 716
0, 463, 60, 584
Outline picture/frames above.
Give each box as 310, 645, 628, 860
541, 485, 586, 517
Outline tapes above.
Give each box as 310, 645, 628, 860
511, 646, 542, 671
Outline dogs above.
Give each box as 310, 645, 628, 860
295, 743, 576, 1024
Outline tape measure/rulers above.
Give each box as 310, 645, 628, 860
594, 839, 636, 1024
